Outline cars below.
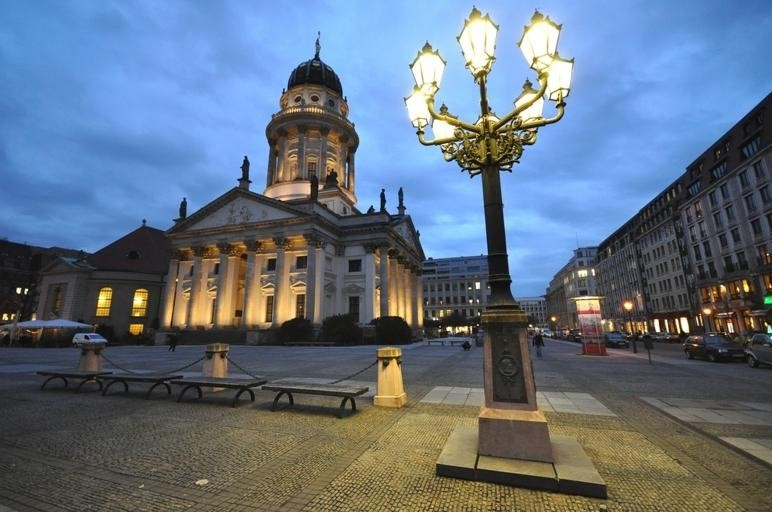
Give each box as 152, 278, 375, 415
639, 332, 680, 343
541, 329, 554, 338
681, 330, 771, 370
558, 328, 629, 349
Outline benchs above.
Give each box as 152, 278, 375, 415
425, 339, 445, 346
262, 379, 370, 412
94, 372, 184, 399
447, 340, 465, 347
34, 368, 115, 391
169, 376, 268, 407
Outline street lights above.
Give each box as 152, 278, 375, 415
623, 301, 638, 354
403, 4, 577, 463
550, 315, 557, 338
7, 283, 31, 345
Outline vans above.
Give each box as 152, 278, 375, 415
71, 333, 109, 349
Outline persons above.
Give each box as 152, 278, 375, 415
531, 327, 546, 358
167, 333, 179, 351
